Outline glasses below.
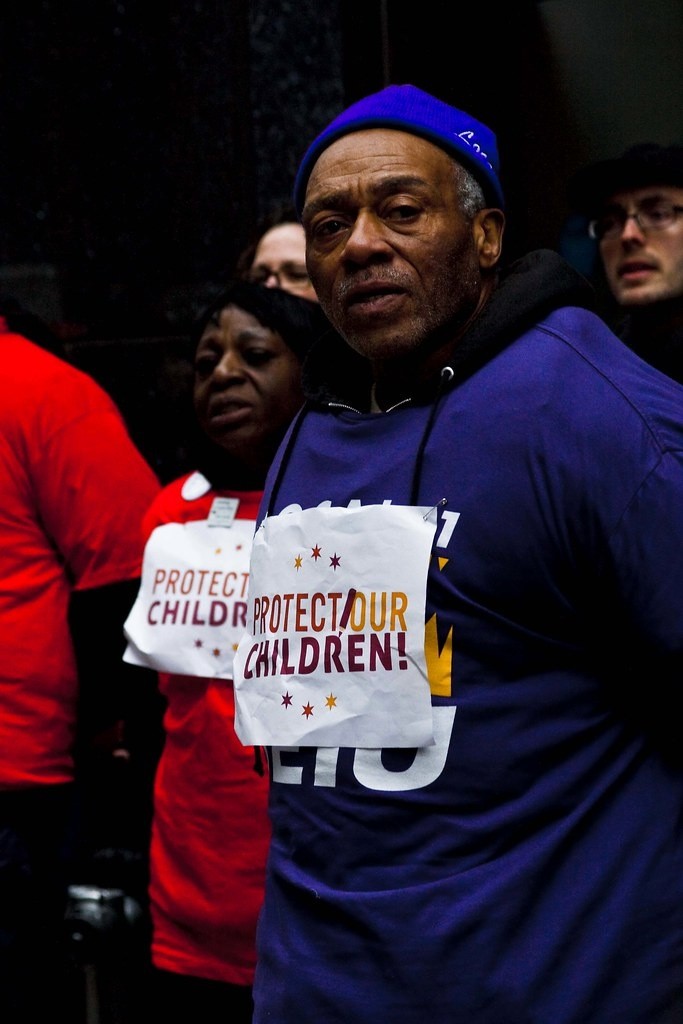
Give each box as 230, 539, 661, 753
589, 202, 682, 239
248, 264, 313, 291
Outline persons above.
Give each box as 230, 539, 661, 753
251, 84, 683, 1024
0, 312, 165, 1024
117, 279, 369, 1024
585, 140, 683, 388
241, 203, 320, 302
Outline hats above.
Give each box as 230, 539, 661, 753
558, 142, 681, 219
292, 83, 502, 226
